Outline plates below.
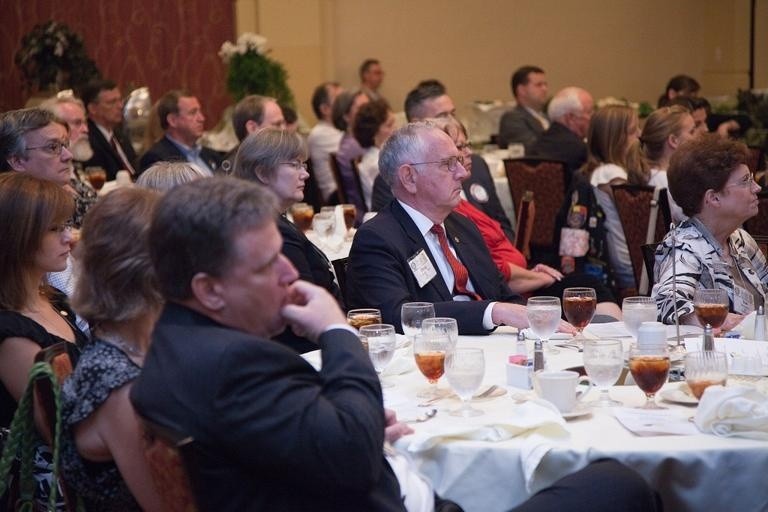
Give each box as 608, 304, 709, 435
514, 401, 596, 421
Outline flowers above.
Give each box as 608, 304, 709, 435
207, 29, 271, 69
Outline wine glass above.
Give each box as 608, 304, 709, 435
582, 342, 730, 410
291, 203, 359, 252
524, 286, 732, 351
345, 303, 484, 412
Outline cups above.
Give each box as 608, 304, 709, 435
85, 166, 134, 191
471, 143, 524, 174
529, 367, 592, 410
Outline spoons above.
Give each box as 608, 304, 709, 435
401, 409, 437, 427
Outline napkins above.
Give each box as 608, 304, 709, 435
693, 383, 766, 438
407, 392, 570, 492
375, 331, 418, 377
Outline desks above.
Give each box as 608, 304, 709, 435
299, 321, 767, 511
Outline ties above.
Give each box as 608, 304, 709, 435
111, 134, 138, 176
429, 223, 484, 301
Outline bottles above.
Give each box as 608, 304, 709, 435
755, 306, 766, 342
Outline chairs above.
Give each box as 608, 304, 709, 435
33, 347, 97, 512
23, 88, 766, 345
134, 411, 217, 511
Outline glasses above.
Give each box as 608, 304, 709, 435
47, 221, 73, 232
278, 158, 308, 171
24, 137, 71, 157
456, 141, 473, 150
722, 171, 756, 191
407, 154, 466, 172
